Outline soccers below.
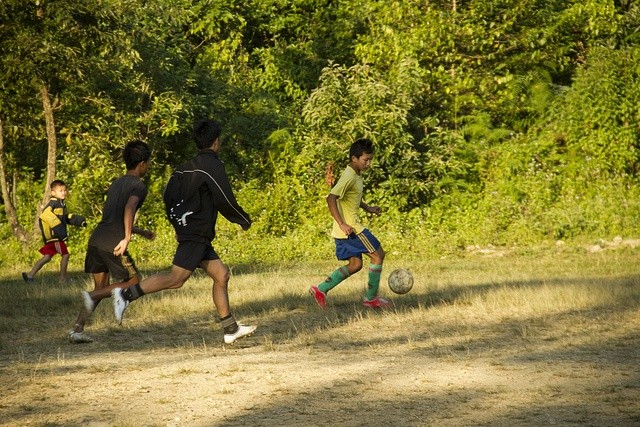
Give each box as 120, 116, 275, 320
389, 269, 413, 293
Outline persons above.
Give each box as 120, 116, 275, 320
309, 138, 392, 310
23, 180, 86, 282
112, 118, 257, 344
66, 140, 150, 342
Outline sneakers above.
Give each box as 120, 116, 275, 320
67, 329, 94, 344
224, 325, 257, 344
111, 288, 129, 326
309, 286, 327, 310
363, 296, 388, 307
81, 291, 94, 315
22, 272, 33, 282
